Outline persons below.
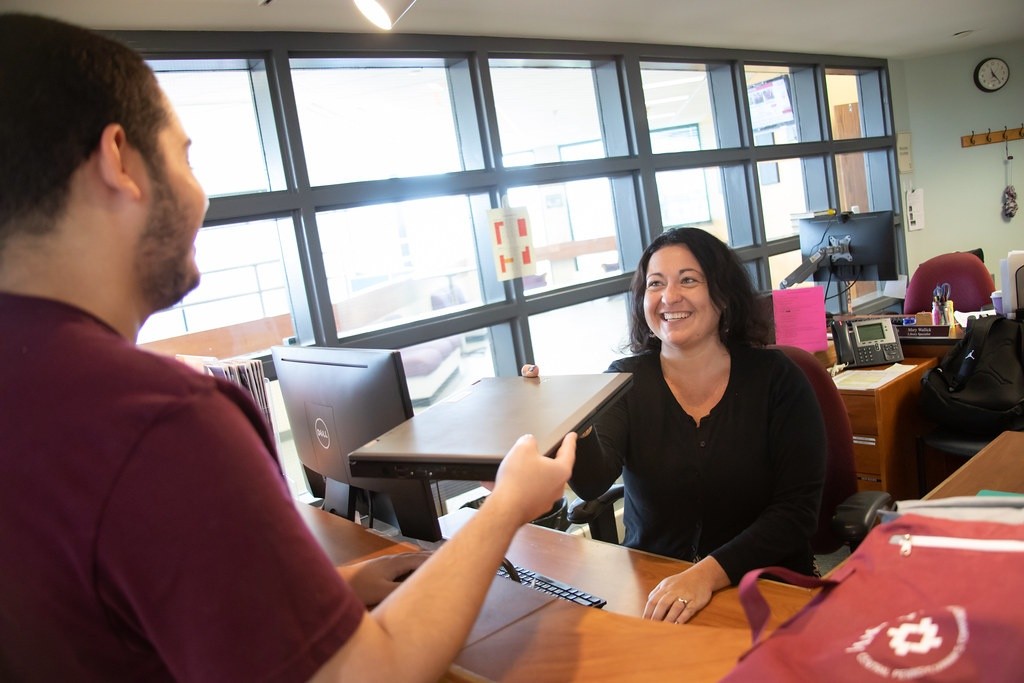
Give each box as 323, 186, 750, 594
0, 12, 576, 683
521, 227, 827, 624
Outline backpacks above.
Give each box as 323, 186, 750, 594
919, 308, 1024, 442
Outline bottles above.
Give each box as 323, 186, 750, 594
902, 318, 916, 325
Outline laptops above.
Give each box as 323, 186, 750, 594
348, 373, 634, 481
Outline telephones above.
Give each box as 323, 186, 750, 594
830, 318, 905, 367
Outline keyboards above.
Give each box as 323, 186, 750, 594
496, 566, 607, 610
826, 317, 915, 326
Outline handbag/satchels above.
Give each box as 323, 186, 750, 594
720, 514, 1024, 683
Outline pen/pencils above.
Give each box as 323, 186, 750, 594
933, 283, 948, 301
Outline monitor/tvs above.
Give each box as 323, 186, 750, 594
271, 345, 443, 543
799, 210, 899, 283
753, 291, 777, 346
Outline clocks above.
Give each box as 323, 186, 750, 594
973, 58, 1010, 93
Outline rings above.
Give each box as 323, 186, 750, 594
677, 598, 687, 606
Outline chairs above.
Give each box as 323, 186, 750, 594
904, 253, 996, 315
750, 344, 891, 555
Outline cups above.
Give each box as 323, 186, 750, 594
990, 296, 1003, 316
930, 300, 955, 337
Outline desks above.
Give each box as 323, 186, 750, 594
790, 314, 988, 494
286, 430, 1024, 683
